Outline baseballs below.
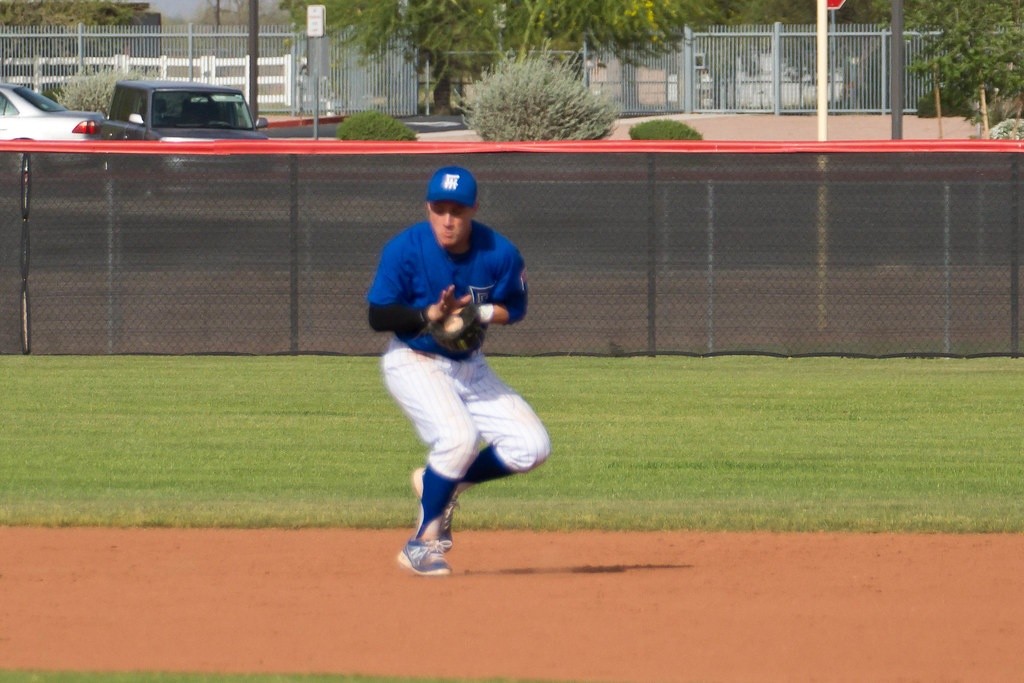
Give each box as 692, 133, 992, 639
444, 314, 464, 332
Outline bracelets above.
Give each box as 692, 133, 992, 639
478, 304, 492, 323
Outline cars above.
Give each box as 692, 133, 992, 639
0, 84, 106, 141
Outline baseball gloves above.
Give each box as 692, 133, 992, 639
430, 306, 486, 353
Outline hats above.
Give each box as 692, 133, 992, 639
426, 165, 478, 207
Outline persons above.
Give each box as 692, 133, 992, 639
368, 166, 551, 574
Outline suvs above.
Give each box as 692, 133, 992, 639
101, 79, 269, 140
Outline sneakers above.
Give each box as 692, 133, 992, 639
412, 467, 459, 553
396, 539, 453, 577
